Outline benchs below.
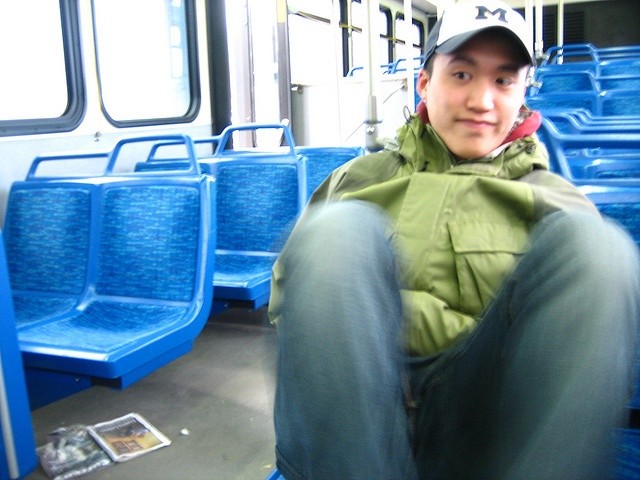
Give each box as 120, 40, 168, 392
523, 37, 640, 253
1, 133, 219, 392
343, 56, 424, 117
266, 427, 639, 479
254, 146, 365, 211
129, 123, 310, 321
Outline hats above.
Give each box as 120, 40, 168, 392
422, 1, 534, 70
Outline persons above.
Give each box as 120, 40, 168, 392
268, 0, 640, 480
